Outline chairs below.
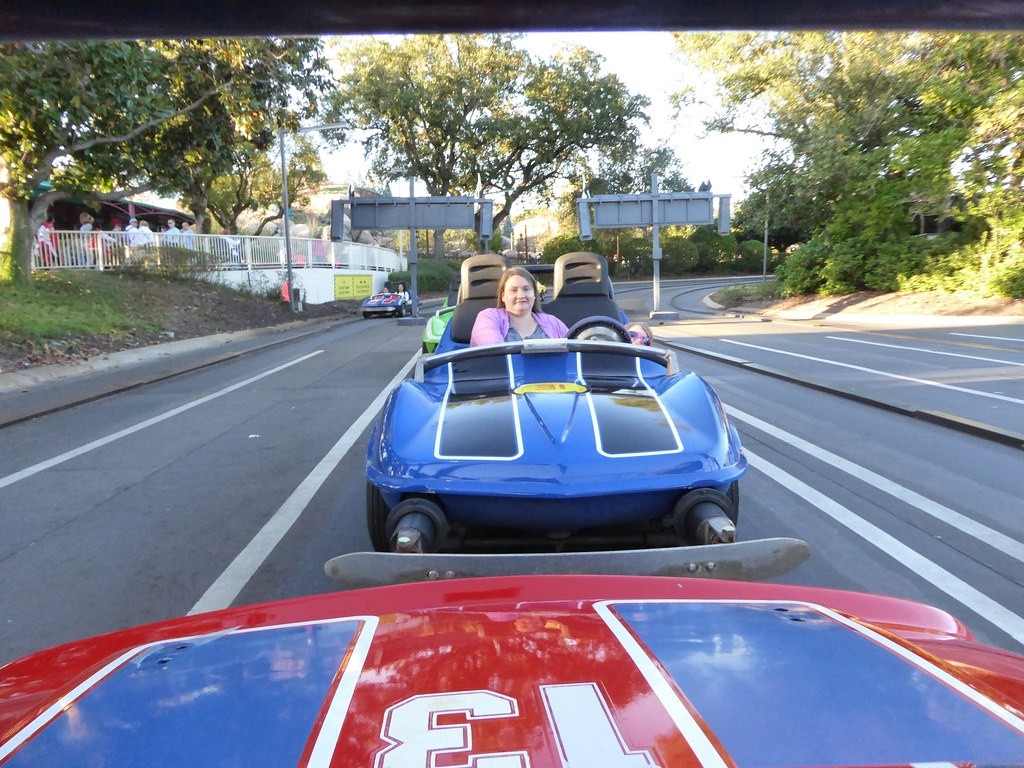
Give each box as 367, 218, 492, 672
541, 253, 626, 330
381, 282, 393, 293
397, 282, 411, 298
437, 253, 507, 356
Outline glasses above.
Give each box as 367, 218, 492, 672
628, 331, 651, 346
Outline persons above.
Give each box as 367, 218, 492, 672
624, 322, 654, 349
382, 284, 410, 302
469, 267, 569, 349
32, 211, 242, 270
